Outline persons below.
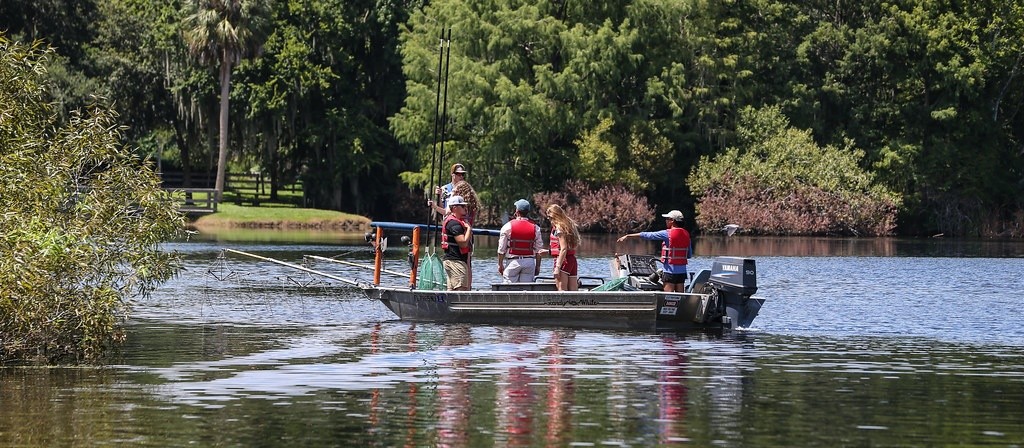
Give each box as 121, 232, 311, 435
435, 163, 468, 218
497, 198, 543, 284
439, 196, 472, 292
617, 209, 690, 293
426, 181, 482, 292
545, 204, 580, 291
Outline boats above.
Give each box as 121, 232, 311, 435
359, 220, 767, 335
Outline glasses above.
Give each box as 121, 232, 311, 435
453, 172, 464, 177
452, 205, 466, 209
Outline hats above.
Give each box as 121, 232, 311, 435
661, 209, 683, 222
449, 196, 468, 205
514, 199, 530, 212
451, 165, 467, 174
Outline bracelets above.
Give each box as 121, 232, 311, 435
434, 206, 437, 212
